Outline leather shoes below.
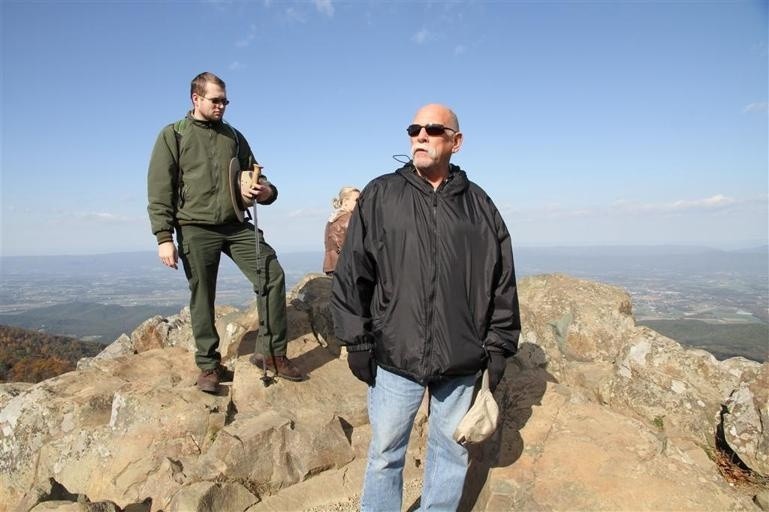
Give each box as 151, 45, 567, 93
252, 351, 305, 383
196, 367, 221, 395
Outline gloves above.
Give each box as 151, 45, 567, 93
485, 350, 507, 393
347, 348, 378, 389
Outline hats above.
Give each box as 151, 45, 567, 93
451, 368, 501, 449
228, 156, 260, 226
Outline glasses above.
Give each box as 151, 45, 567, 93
198, 95, 229, 105
406, 123, 457, 137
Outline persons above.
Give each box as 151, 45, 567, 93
147, 73, 304, 395
323, 186, 362, 276
330, 102, 522, 512
